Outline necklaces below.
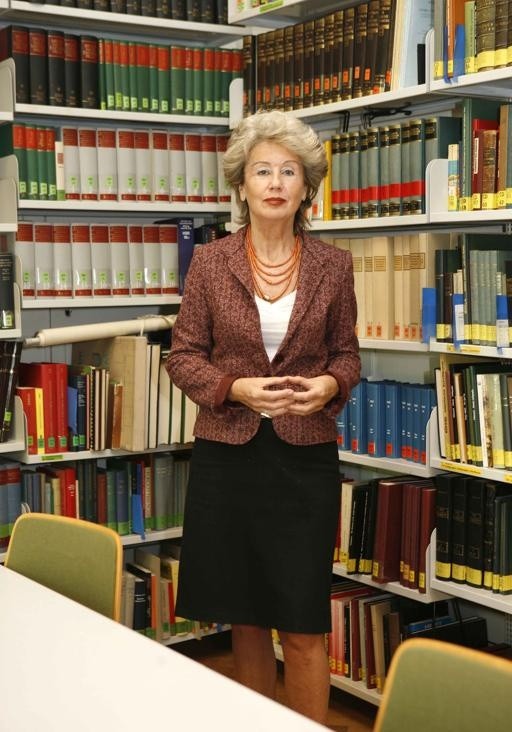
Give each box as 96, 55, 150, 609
248, 241, 298, 286
247, 229, 299, 276
248, 235, 302, 302
248, 224, 298, 267
246, 243, 302, 303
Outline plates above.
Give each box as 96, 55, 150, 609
417, 1, 511, 626
1, 3, 250, 653
235, 1, 432, 709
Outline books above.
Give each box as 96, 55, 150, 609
319, 232, 511, 349
2, 122, 234, 201
272, 581, 510, 695
121, 538, 225, 643
241, 3, 510, 119
241, 0, 510, 697
335, 353, 510, 469
1, 253, 15, 329
303, 106, 509, 222
16, 218, 230, 301
1, 1, 241, 119
1, 455, 192, 554
417, 44, 425, 85
1, 335, 201, 456
333, 474, 509, 593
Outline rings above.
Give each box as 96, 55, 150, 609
260, 409, 272, 419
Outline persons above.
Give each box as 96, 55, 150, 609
164, 108, 361, 725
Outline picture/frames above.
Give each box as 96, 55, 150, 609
1, 556, 338, 732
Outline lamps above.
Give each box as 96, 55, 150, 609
370, 631, 511, 732
5, 505, 131, 624
